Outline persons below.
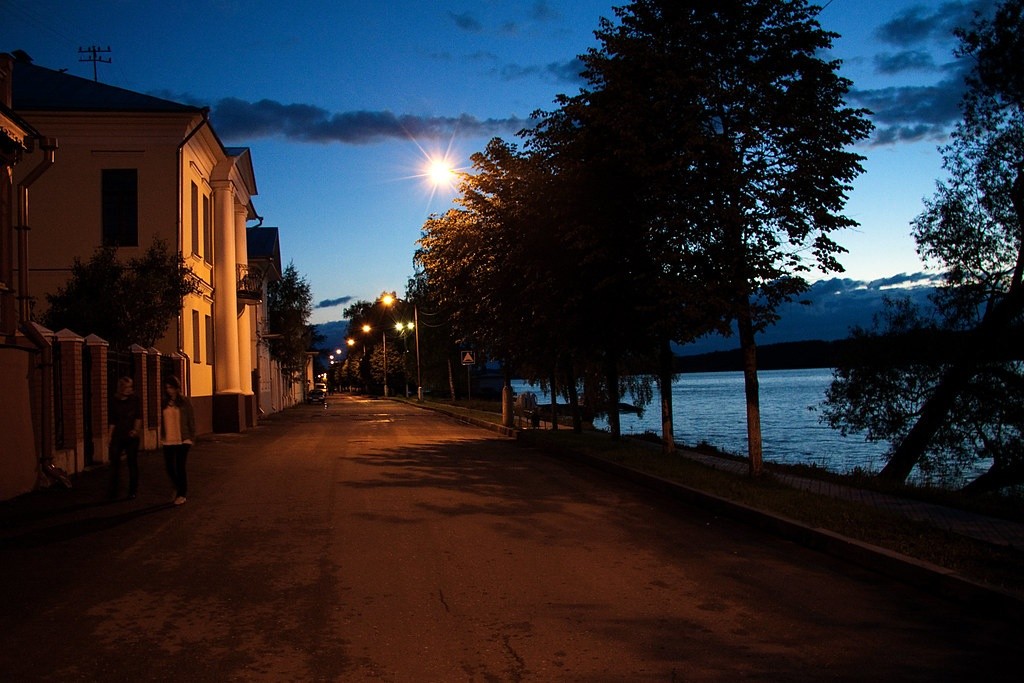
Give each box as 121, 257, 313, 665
161, 376, 196, 505
106, 377, 142, 502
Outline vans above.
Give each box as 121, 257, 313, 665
315, 383, 327, 395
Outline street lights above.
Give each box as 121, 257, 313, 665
393, 322, 415, 398
381, 295, 424, 401
363, 324, 391, 396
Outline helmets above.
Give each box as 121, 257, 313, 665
117, 376, 135, 396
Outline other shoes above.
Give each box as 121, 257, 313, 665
175, 497, 187, 504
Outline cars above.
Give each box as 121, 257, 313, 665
307, 390, 325, 404
